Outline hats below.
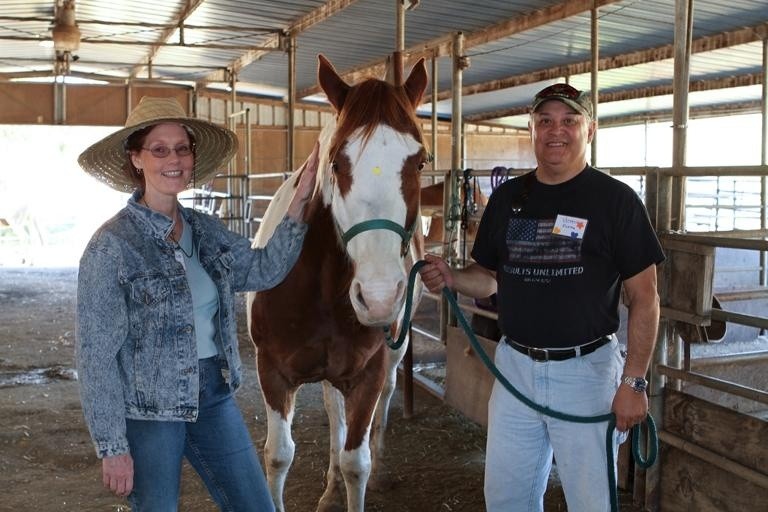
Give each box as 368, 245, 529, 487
78, 94, 239, 193
529, 83, 594, 124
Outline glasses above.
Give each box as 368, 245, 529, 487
139, 142, 197, 158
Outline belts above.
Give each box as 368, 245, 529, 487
504, 335, 612, 363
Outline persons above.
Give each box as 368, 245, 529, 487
419, 82, 667, 511
73, 95, 321, 512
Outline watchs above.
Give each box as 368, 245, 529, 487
621, 374, 649, 392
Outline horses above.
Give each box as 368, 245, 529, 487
415, 181, 490, 244
245, 51, 428, 512
